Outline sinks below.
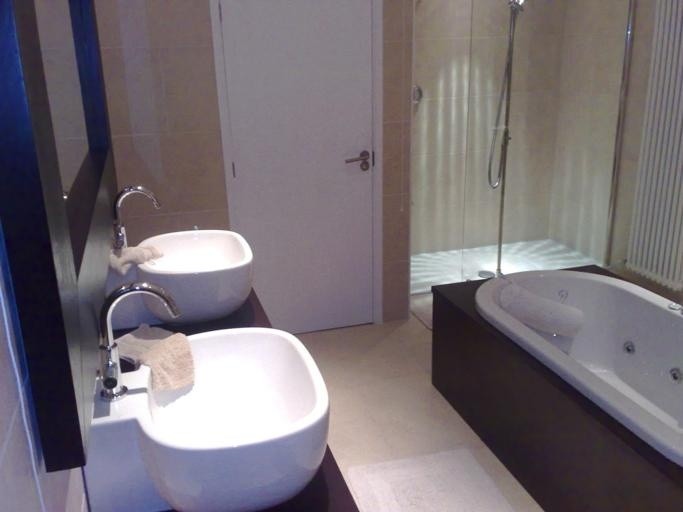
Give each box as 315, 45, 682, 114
105, 229, 253, 333
81, 325, 330, 512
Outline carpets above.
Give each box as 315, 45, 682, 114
344, 445, 515, 512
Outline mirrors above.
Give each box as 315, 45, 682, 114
0, 0, 122, 470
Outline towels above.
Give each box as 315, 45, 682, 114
109, 247, 195, 394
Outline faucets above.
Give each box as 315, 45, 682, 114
113, 184, 164, 249
98, 280, 183, 401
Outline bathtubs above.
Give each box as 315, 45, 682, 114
475, 268, 682, 466
